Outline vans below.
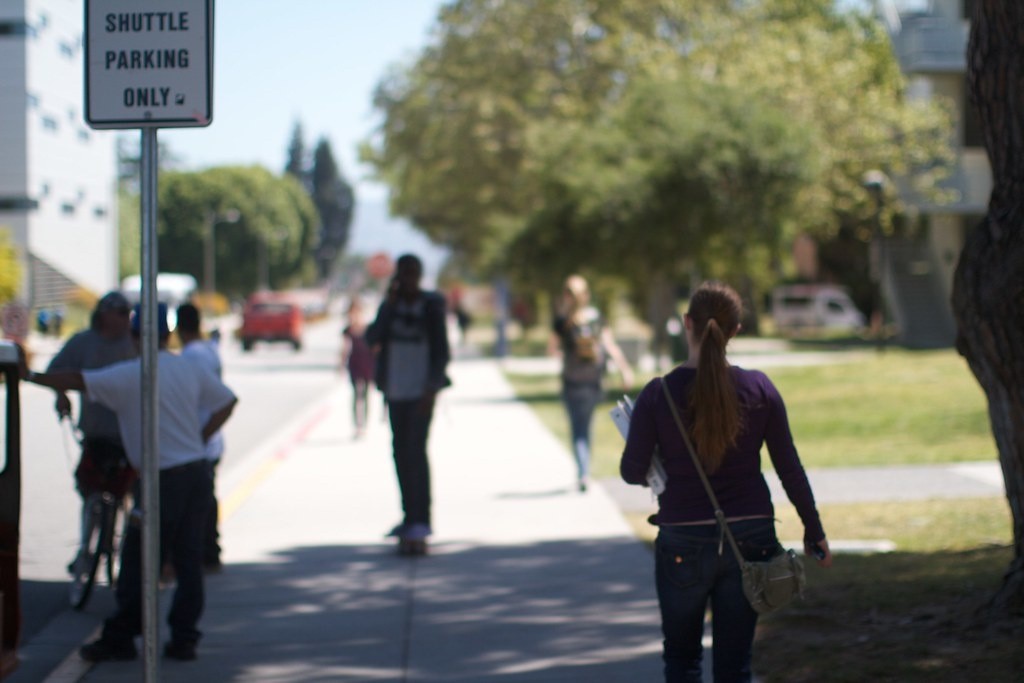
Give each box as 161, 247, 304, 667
120, 276, 196, 330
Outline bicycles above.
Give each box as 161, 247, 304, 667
58, 409, 131, 611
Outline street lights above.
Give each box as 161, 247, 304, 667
865, 173, 890, 355
206, 211, 243, 303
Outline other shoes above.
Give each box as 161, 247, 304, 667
68, 550, 94, 575
164, 640, 196, 660
80, 639, 136, 662
400, 525, 428, 556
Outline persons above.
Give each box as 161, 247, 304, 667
176, 302, 224, 574
620, 282, 830, 683
46, 294, 138, 574
363, 255, 452, 558
552, 275, 637, 491
343, 295, 373, 432
12, 302, 238, 662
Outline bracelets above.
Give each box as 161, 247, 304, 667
28, 371, 36, 379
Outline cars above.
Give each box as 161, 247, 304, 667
241, 293, 303, 352
774, 284, 863, 334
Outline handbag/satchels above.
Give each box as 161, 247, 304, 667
741, 549, 806, 615
